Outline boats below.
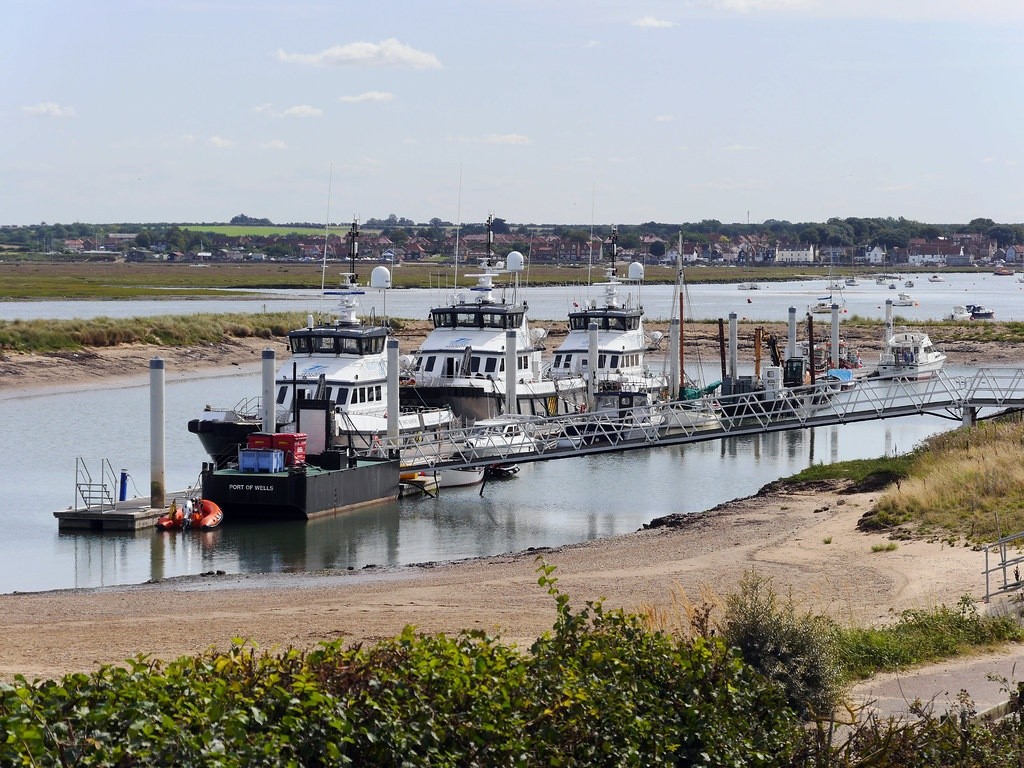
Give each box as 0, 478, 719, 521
155, 497, 224, 533
943, 304, 972, 323
966, 303, 995, 319
994, 266, 1016, 275
1018, 276, 1024, 283
927, 274, 945, 282
185, 159, 861, 521
891, 292, 918, 306
877, 331, 946, 381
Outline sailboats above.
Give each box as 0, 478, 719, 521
905, 264, 914, 287
875, 248, 897, 290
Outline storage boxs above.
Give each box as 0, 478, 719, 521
321, 450, 347, 470
236, 443, 284, 473
305, 454, 332, 467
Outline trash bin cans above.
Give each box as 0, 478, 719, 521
272, 433, 307, 465
246, 432, 272, 449
